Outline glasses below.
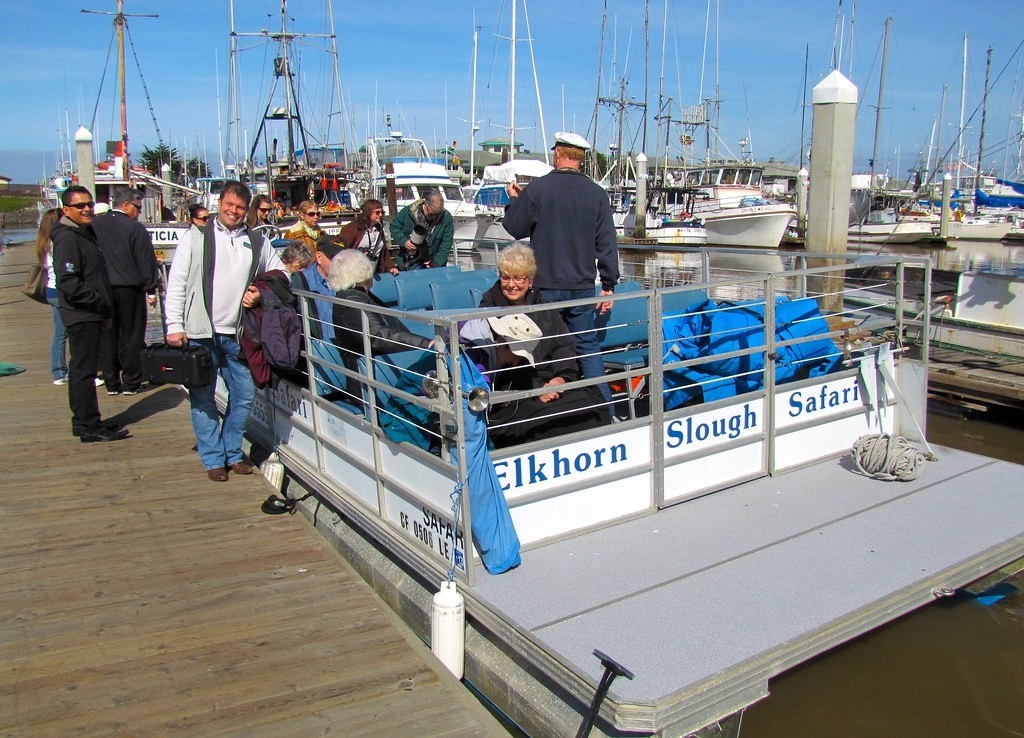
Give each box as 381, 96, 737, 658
258, 207, 272, 212
67, 201, 94, 209
426, 206, 441, 217
500, 276, 528, 284
131, 202, 142, 211
195, 216, 210, 222
289, 263, 308, 271
302, 212, 320, 217
373, 210, 386, 215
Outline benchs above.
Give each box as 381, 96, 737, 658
312, 265, 708, 419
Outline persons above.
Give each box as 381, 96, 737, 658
379, 312, 543, 456
36, 207, 105, 387
329, 248, 435, 404
246, 194, 275, 240
281, 241, 314, 272
303, 233, 347, 339
189, 204, 210, 227
90, 188, 161, 396
337, 200, 400, 275
389, 189, 454, 271
49, 185, 130, 442
502, 131, 620, 423
284, 200, 328, 270
165, 180, 291, 481
479, 242, 611, 450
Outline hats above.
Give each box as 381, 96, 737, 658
550, 132, 591, 150
94, 203, 112, 215
316, 235, 346, 257
486, 313, 543, 368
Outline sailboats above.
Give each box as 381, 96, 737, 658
34, 0, 1024, 391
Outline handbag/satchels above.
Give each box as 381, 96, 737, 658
21, 265, 50, 304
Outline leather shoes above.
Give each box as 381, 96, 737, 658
73, 422, 119, 436
80, 430, 129, 442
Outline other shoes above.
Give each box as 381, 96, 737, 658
208, 467, 228, 481
108, 391, 119, 395
94, 378, 105, 387
54, 374, 68, 385
231, 462, 252, 474
122, 386, 142, 395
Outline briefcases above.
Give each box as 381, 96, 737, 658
143, 338, 212, 389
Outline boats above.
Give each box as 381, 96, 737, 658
181, 234, 1023, 737
